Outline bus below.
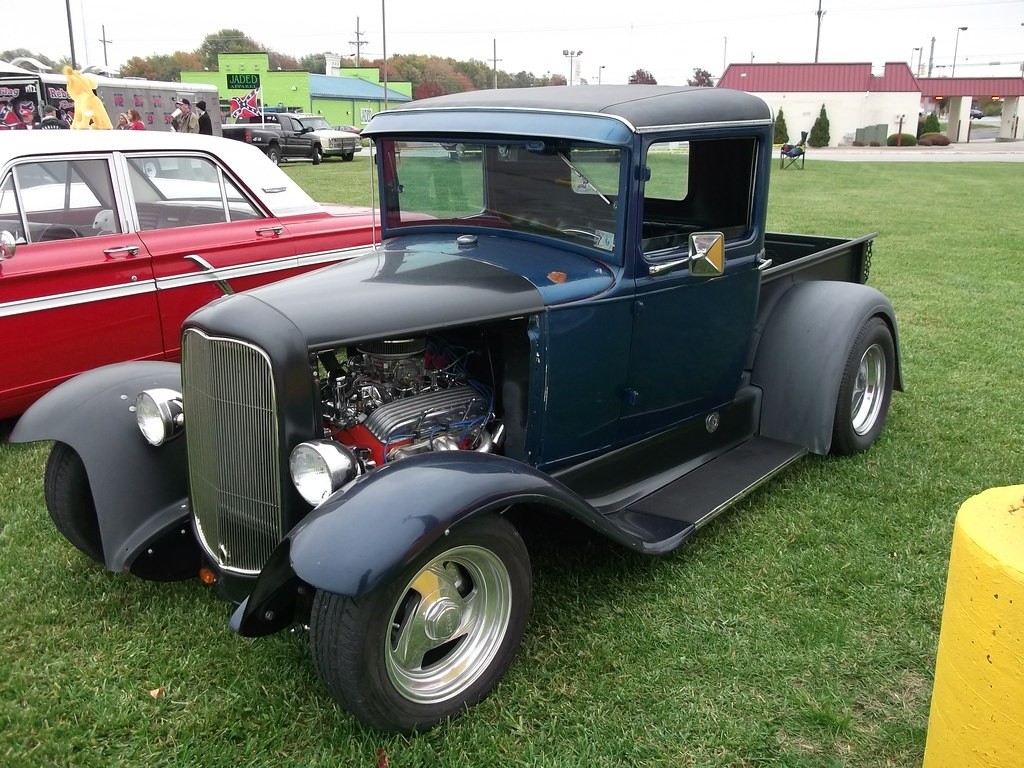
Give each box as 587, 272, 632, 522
0, 55, 223, 140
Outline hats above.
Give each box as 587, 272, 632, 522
196, 101, 206, 111
44, 105, 59, 114
176, 99, 190, 105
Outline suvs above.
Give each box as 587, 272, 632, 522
255, 112, 362, 164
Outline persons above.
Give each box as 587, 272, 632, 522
116, 110, 144, 130
16, 109, 35, 130
195, 101, 212, 135
170, 98, 199, 134
32, 105, 68, 129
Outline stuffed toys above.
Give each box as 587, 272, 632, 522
62, 66, 113, 129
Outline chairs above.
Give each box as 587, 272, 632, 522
779, 131, 808, 169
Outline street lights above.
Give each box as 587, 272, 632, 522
952, 26, 968, 77
562, 50, 583, 86
599, 65, 605, 85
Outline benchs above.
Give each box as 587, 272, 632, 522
133, 202, 257, 231
561, 209, 704, 252
0, 218, 116, 247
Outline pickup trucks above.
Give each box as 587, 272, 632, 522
220, 112, 324, 169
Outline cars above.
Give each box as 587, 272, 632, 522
332, 125, 365, 134
0, 129, 513, 422
8, 77, 906, 742
969, 109, 984, 120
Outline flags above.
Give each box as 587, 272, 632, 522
228, 87, 261, 119
